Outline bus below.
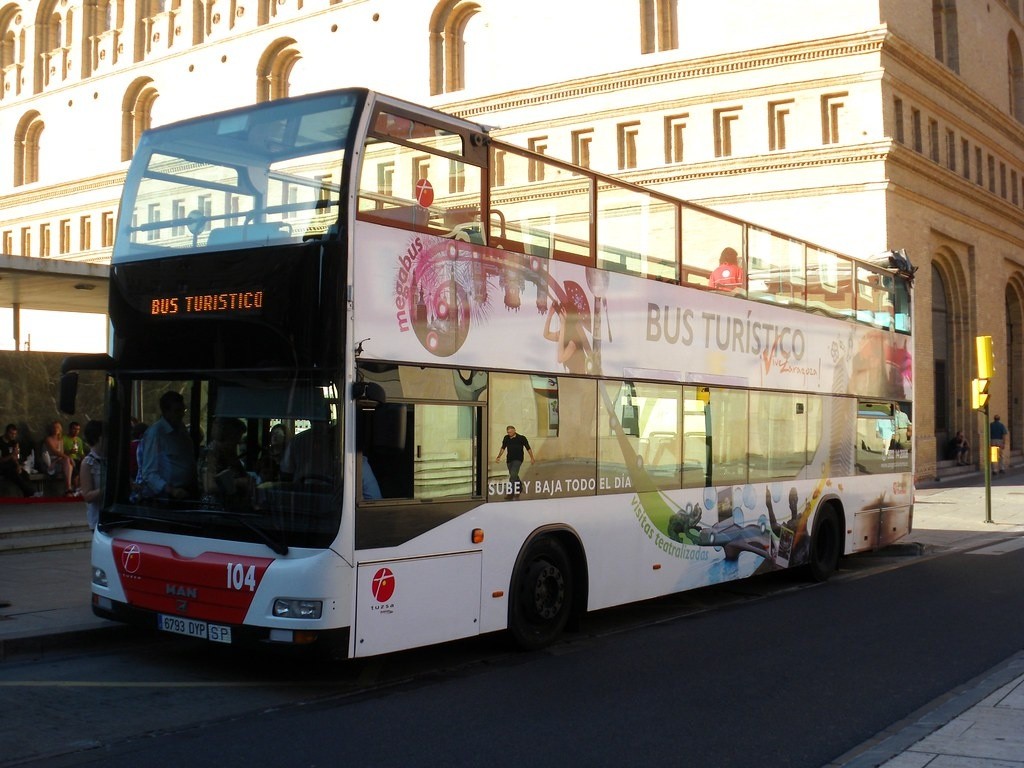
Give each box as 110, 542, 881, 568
57, 85, 917, 667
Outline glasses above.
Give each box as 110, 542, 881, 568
176, 408, 188, 413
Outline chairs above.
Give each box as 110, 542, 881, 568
646, 428, 707, 491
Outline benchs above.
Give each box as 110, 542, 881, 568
356, 204, 433, 227
0, 473, 78, 501
205, 218, 291, 245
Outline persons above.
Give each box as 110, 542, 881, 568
0, 419, 104, 532
708, 247, 743, 291
128, 390, 270, 508
270, 402, 383, 500
495, 425, 535, 500
989, 415, 1008, 475
950, 430, 971, 466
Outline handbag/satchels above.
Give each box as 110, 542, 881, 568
42, 451, 51, 468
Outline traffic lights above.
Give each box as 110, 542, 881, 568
972, 377, 990, 411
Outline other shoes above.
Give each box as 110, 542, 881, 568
956, 462, 971, 466
29, 492, 43, 498
999, 470, 1006, 474
516, 489, 523, 497
504, 496, 513, 499
991, 471, 998, 476
63, 490, 76, 498
